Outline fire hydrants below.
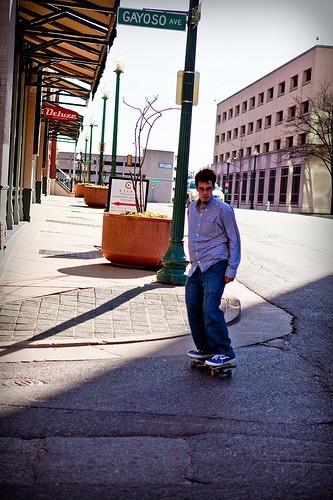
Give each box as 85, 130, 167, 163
265, 201, 271, 211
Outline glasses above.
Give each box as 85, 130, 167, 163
196, 186, 213, 192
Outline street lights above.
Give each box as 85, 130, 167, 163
88, 121, 95, 183
107, 60, 124, 210
225, 158, 232, 204
76, 154, 81, 184
97, 88, 110, 185
84, 135, 89, 183
250, 149, 259, 209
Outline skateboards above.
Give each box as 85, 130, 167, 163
190, 360, 239, 379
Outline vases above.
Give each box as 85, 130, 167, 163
83, 184, 109, 207
101, 212, 173, 270
74, 183, 90, 196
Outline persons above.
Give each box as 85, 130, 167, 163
185, 168, 241, 366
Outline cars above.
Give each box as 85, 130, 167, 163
186, 179, 224, 207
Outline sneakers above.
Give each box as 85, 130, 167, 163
187, 349, 216, 358
205, 353, 237, 366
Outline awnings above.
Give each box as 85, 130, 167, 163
22, 2, 118, 140
